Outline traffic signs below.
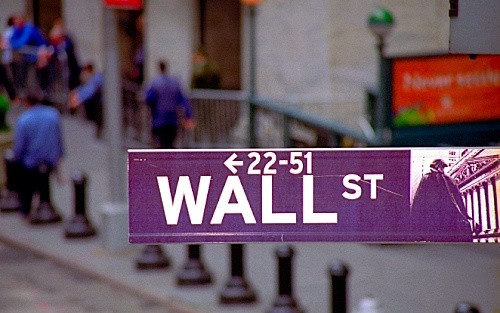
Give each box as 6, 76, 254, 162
128, 147, 500, 244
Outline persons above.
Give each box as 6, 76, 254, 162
145, 63, 195, 148
9, 86, 62, 220
67, 63, 102, 137
0, 15, 79, 113
